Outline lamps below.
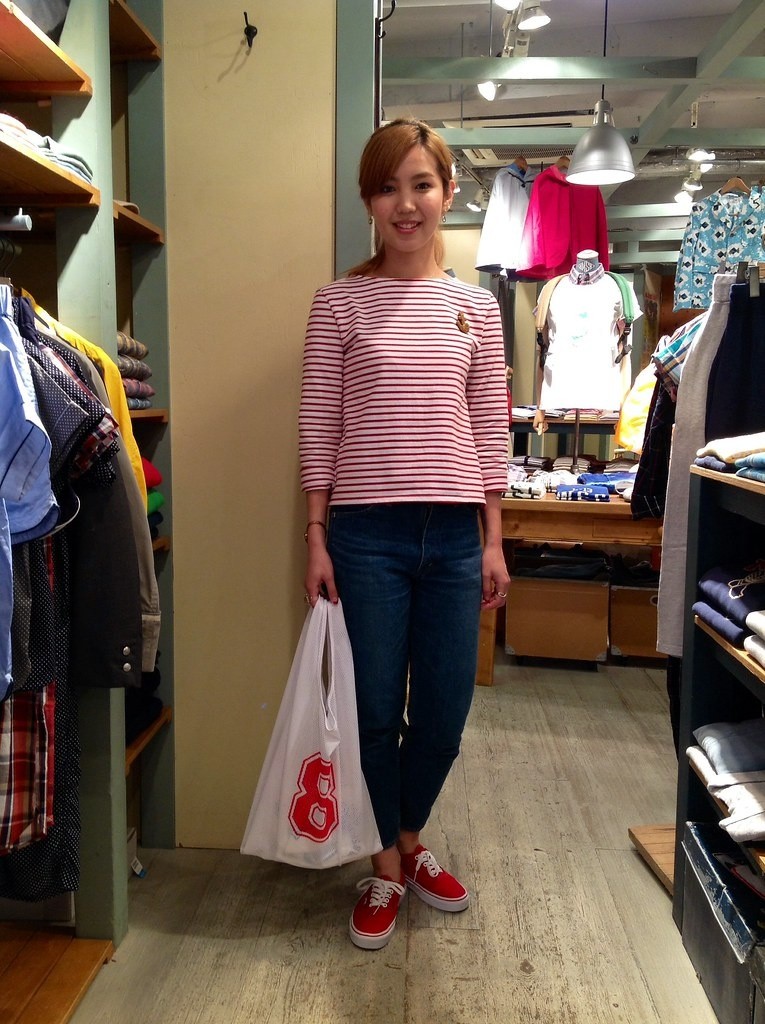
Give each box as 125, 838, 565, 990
564, 0, 636, 188
466, 185, 491, 213
673, 148, 715, 205
517, 0, 551, 31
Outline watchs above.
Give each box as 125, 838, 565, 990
303, 520, 327, 544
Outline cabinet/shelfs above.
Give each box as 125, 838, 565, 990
1, 0, 181, 1024
669, 463, 765, 1024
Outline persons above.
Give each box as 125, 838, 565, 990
295, 115, 513, 955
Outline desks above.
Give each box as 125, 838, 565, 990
476, 494, 664, 686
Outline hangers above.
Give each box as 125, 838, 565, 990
717, 259, 765, 297
514, 150, 529, 172
719, 157, 754, 215
556, 150, 570, 170
0, 238, 24, 300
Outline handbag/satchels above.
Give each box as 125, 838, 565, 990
240, 597, 384, 867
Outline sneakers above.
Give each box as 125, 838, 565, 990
403, 844, 470, 913
349, 863, 406, 950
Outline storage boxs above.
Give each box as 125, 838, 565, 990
680, 819, 765, 1024
611, 582, 668, 660
503, 574, 610, 662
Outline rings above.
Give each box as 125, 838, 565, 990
495, 590, 509, 598
